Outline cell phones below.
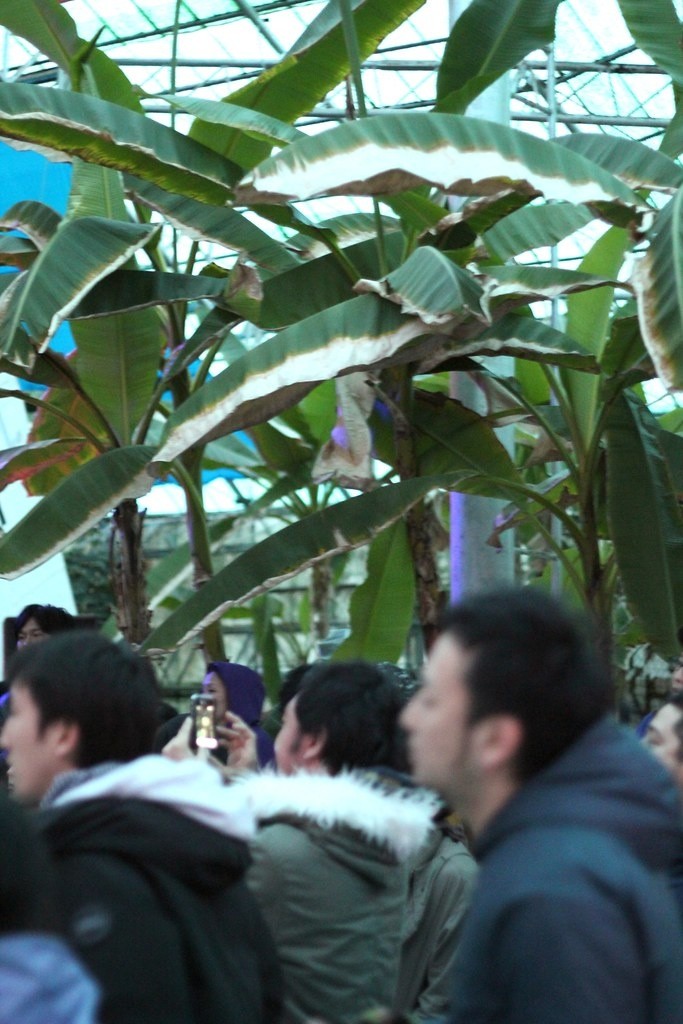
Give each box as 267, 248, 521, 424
189, 693, 220, 751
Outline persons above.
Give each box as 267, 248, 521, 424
1, 604, 77, 764
0, 630, 286, 1024
397, 584, 683, 1024
0, 797, 103, 1024
159, 661, 683, 1024
